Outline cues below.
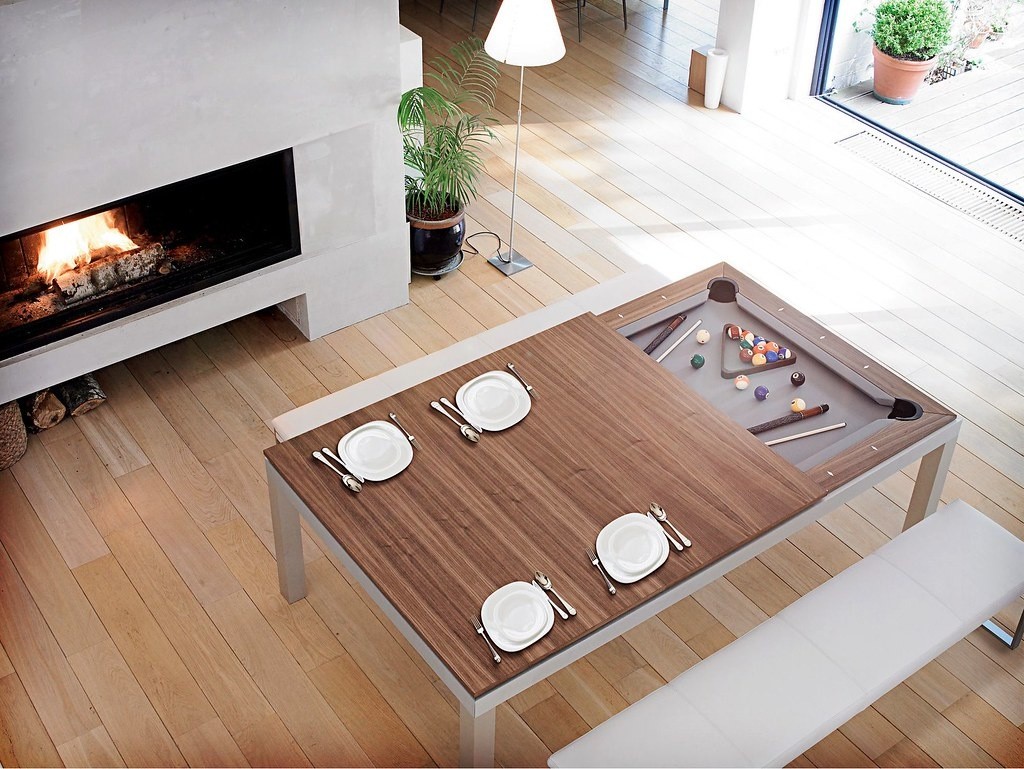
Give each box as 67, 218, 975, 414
655, 320, 702, 366
764, 422, 847, 446
642, 313, 687, 355
747, 403, 830, 434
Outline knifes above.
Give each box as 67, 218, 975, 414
647, 511, 684, 551
321, 447, 364, 484
439, 397, 483, 433
532, 580, 569, 619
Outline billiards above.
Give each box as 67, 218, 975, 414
690, 323, 805, 412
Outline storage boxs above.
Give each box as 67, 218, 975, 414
688, 44, 714, 96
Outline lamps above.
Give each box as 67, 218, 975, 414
483, 0, 566, 275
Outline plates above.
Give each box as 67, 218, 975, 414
480, 581, 554, 653
337, 421, 413, 482
595, 513, 670, 584
455, 369, 532, 432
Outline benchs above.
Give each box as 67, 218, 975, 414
546, 497, 1023, 768
271, 264, 675, 446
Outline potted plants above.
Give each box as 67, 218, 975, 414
396, 37, 502, 278
962, 1, 990, 49
986, 1, 1009, 42
853, 1, 953, 107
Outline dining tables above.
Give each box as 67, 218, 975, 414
260, 260, 963, 769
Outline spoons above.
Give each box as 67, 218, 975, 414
431, 401, 480, 442
312, 451, 362, 492
535, 571, 576, 615
649, 501, 692, 547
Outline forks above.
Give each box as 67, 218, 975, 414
469, 615, 502, 663
585, 546, 616, 594
389, 412, 422, 451
507, 362, 541, 401
